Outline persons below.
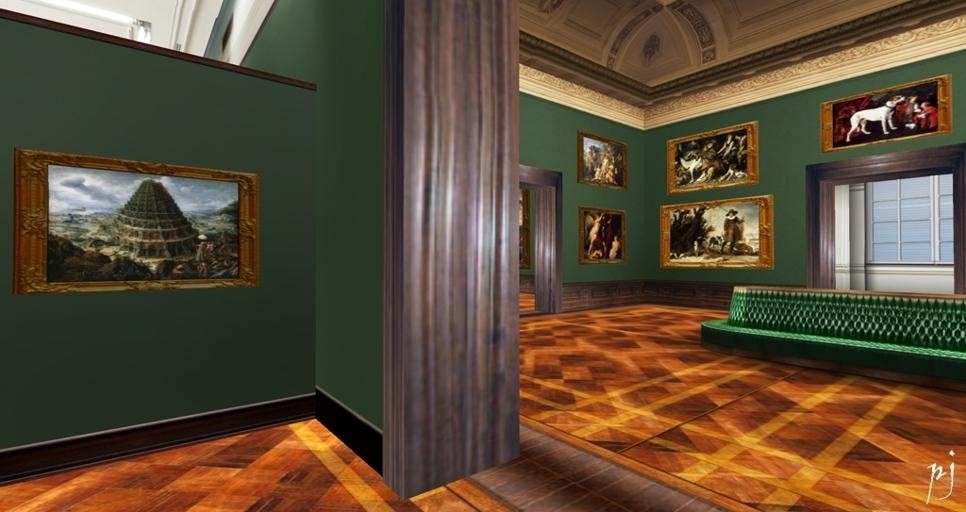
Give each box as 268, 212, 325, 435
716, 134, 747, 181
905, 100, 939, 131
900, 95, 922, 128
717, 208, 745, 255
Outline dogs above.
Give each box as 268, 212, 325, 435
844, 94, 907, 143
